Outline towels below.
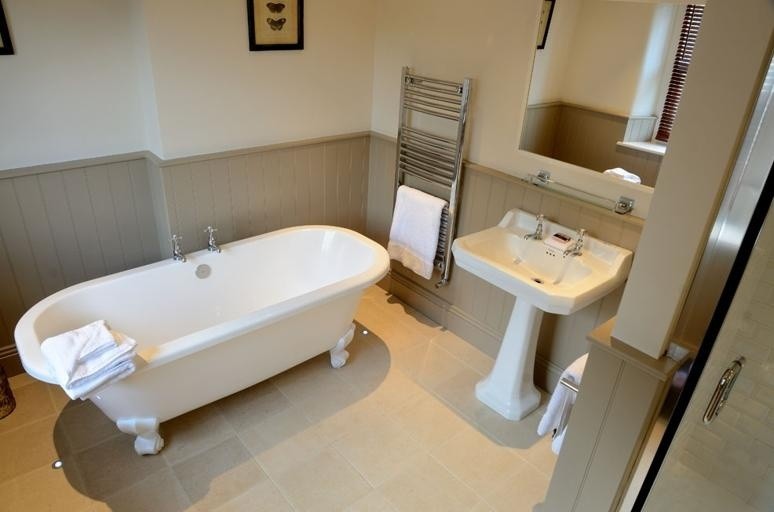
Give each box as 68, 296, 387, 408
537, 351, 586, 455
387, 185, 448, 280
603, 167, 641, 185
40, 319, 139, 403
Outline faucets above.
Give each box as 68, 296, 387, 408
168, 234, 185, 263
562, 228, 587, 258
522, 212, 545, 242
203, 225, 222, 254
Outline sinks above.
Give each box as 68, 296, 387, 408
450, 207, 634, 316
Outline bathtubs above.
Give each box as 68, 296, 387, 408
14, 223, 392, 456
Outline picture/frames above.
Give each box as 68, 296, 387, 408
535, 0, 556, 49
246, 0, 304, 52
0, 1, 14, 55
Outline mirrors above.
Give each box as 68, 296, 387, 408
517, 0, 708, 190
